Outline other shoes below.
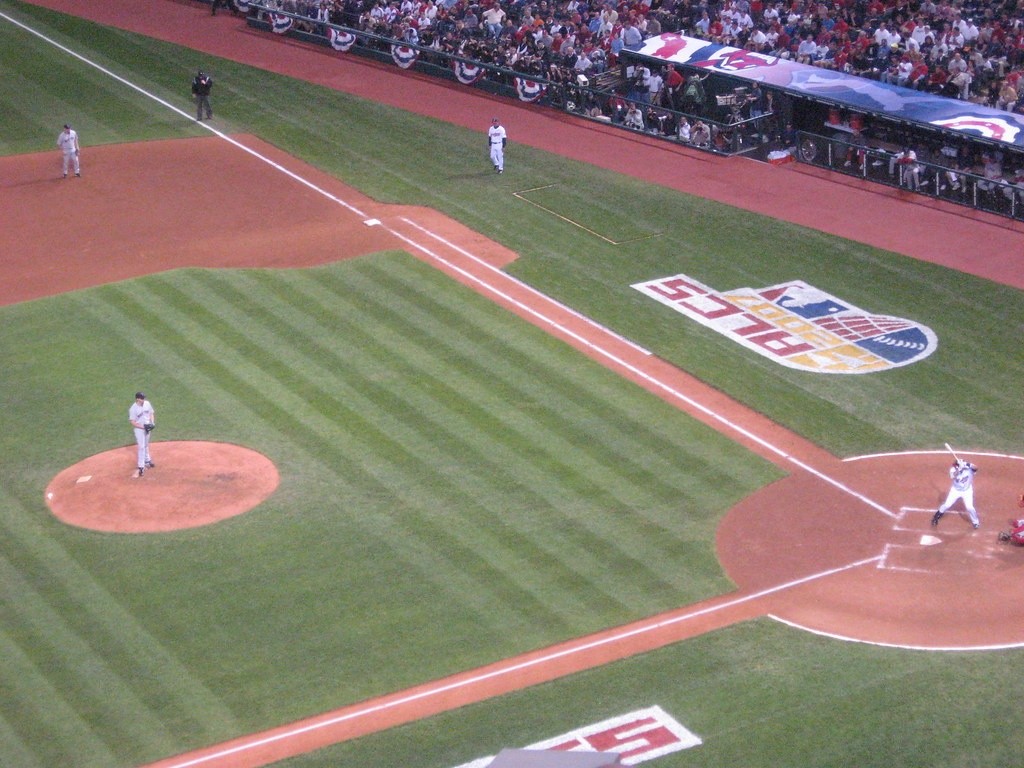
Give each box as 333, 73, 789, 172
933, 518, 939, 526
499, 170, 502, 175
145, 461, 154, 467
974, 523, 980, 528
494, 165, 498, 171
197, 118, 202, 121
207, 116, 212, 119
63, 174, 67, 178
75, 173, 81, 176
139, 468, 144, 475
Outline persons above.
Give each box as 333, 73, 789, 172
57, 124, 81, 178
210, 0, 1024, 219
129, 392, 156, 476
192, 70, 212, 121
932, 460, 981, 528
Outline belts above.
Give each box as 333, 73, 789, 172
492, 142, 501, 144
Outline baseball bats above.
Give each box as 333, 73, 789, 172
944, 442, 964, 468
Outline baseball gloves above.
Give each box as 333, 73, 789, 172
998, 531, 1009, 541
75, 149, 81, 156
144, 423, 155, 436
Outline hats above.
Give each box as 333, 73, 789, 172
136, 393, 145, 399
199, 71, 204, 76
492, 118, 500, 124
64, 125, 70, 129
955, 459, 963, 467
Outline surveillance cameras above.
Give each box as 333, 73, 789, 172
577, 74, 588, 86
